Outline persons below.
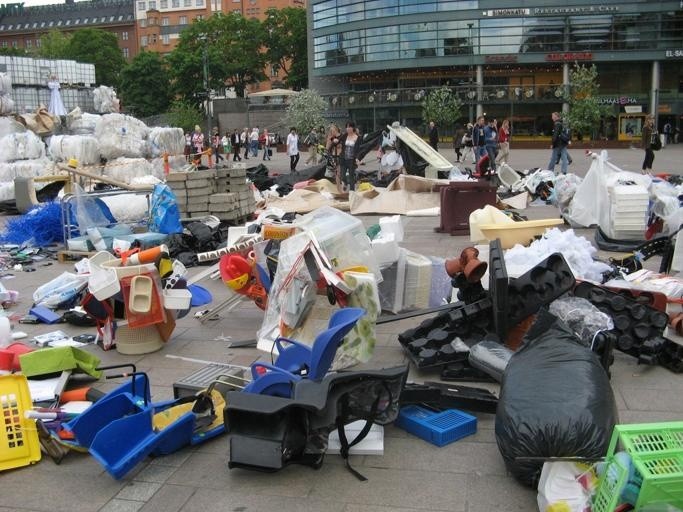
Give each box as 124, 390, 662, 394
48, 74, 67, 115
429, 120, 439, 151
286, 127, 300, 173
641, 114, 657, 174
556, 150, 572, 165
184, 124, 271, 165
548, 112, 568, 175
304, 119, 361, 192
453, 116, 512, 173
663, 121, 671, 144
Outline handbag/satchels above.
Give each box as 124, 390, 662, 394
268, 149, 272, 156
652, 130, 662, 150
303, 134, 314, 147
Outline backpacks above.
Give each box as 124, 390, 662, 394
560, 127, 572, 143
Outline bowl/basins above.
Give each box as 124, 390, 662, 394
476, 218, 566, 248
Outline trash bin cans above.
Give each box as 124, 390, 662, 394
659, 133, 667, 148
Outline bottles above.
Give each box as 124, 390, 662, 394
128, 244, 169, 265
61, 159, 77, 177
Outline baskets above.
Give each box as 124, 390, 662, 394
590, 420, 683, 508
